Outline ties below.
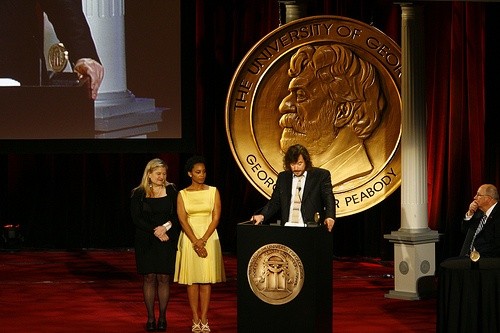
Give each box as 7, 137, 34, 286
469, 214, 487, 252
292, 175, 303, 223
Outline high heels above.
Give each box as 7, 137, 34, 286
191, 318, 211, 333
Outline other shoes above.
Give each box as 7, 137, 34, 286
146, 319, 156, 332
157, 320, 167, 332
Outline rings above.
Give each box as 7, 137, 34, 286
196, 248, 198, 251
202, 254, 204, 255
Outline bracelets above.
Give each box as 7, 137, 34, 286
163, 222, 171, 231
201, 234, 209, 246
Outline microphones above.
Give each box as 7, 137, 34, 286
298, 187, 303, 203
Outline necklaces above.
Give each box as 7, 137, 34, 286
152, 186, 160, 197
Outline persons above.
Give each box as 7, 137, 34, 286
249, 144, 336, 232
128, 158, 178, 331
173, 159, 225, 333
0, 0, 105, 99
460, 183, 500, 263
